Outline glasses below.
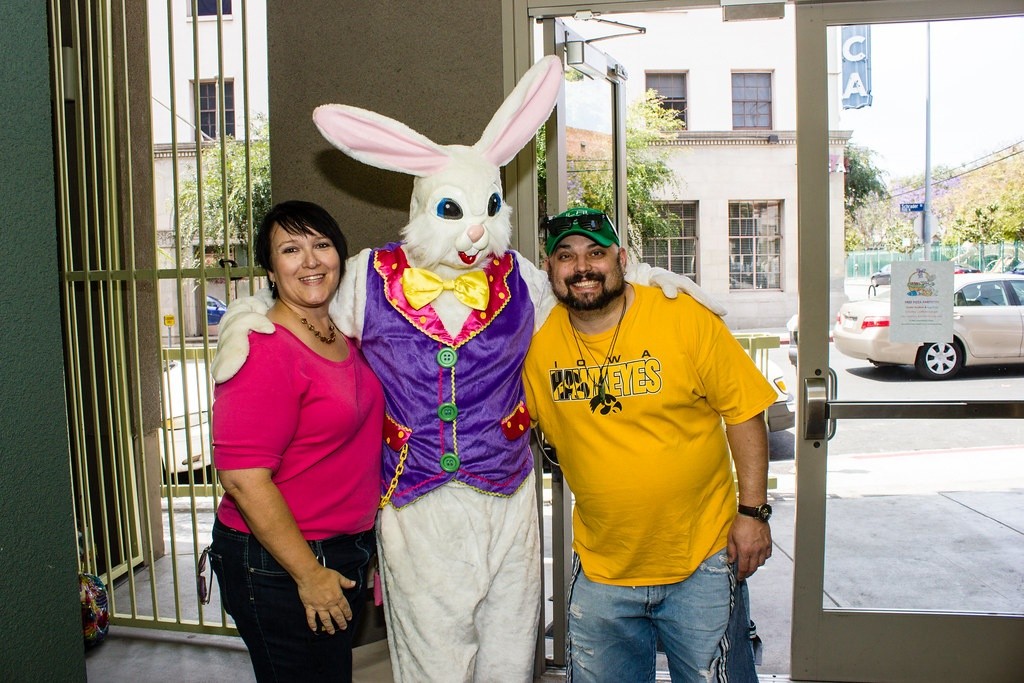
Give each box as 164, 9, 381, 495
542, 213, 606, 236
197, 545, 214, 605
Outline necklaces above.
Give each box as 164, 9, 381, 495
567, 290, 626, 403
289, 308, 338, 344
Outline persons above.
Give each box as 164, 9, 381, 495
521, 207, 779, 683
212, 201, 387, 683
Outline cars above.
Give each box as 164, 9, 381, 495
205, 294, 227, 326
954, 263, 981, 274
834, 272, 1024, 382
1005, 261, 1024, 275
870, 263, 891, 287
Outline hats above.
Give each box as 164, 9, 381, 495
545, 207, 620, 258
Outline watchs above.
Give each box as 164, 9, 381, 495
737, 502, 772, 523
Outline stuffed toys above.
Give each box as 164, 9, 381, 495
212, 58, 729, 683
